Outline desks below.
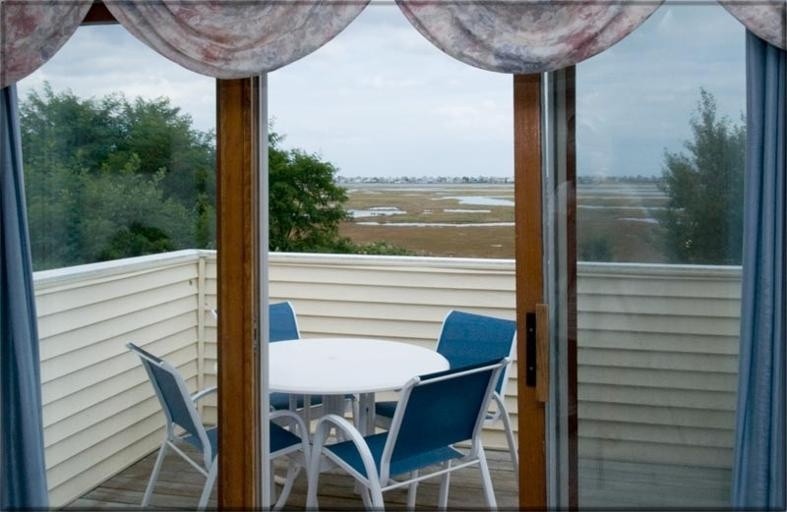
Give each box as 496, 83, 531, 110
255, 334, 457, 511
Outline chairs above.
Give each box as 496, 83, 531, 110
308, 353, 512, 510
369, 306, 519, 482
207, 297, 362, 498
122, 339, 313, 511
